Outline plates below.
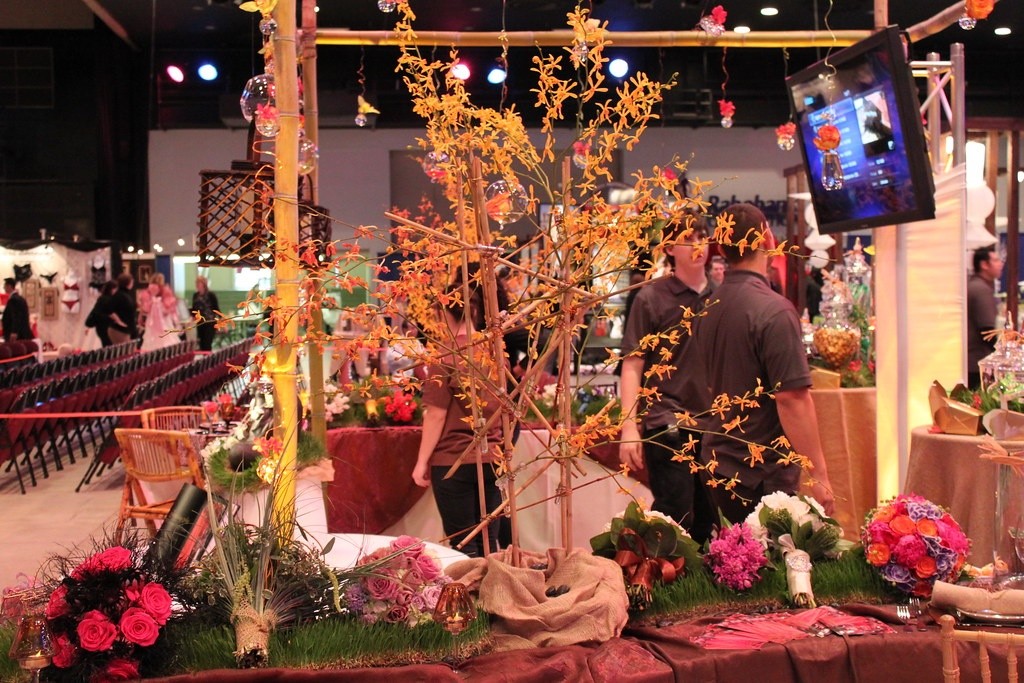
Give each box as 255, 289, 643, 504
955, 608, 1024, 625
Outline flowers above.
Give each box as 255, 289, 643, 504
744, 488, 843, 607
43, 545, 173, 682
589, 501, 702, 610
169, 525, 430, 668
706, 521, 770, 588
335, 534, 453, 628
860, 491, 968, 593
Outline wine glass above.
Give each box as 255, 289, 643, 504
9, 616, 61, 683
432, 583, 478, 677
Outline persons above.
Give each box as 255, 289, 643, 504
1, 278, 36, 343
85, 273, 134, 347
189, 276, 228, 354
88, 256, 110, 296
386, 318, 427, 375
60, 271, 83, 314
618, 207, 721, 552
804, 264, 847, 323
691, 201, 835, 533
138, 273, 183, 350
411, 283, 512, 556
968, 246, 1004, 392
709, 256, 726, 285
456, 261, 536, 364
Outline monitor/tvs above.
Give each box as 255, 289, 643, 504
786, 24, 935, 236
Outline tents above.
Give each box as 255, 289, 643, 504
0, 237, 112, 344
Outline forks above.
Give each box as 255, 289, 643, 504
894, 597, 925, 634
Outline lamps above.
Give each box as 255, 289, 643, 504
165, 1, 1023, 223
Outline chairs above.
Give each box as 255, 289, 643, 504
939, 614, 1024, 683
114, 428, 223, 546
0, 332, 264, 495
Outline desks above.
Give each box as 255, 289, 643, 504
807, 386, 877, 541
905, 425, 1024, 575
306, 421, 657, 556
